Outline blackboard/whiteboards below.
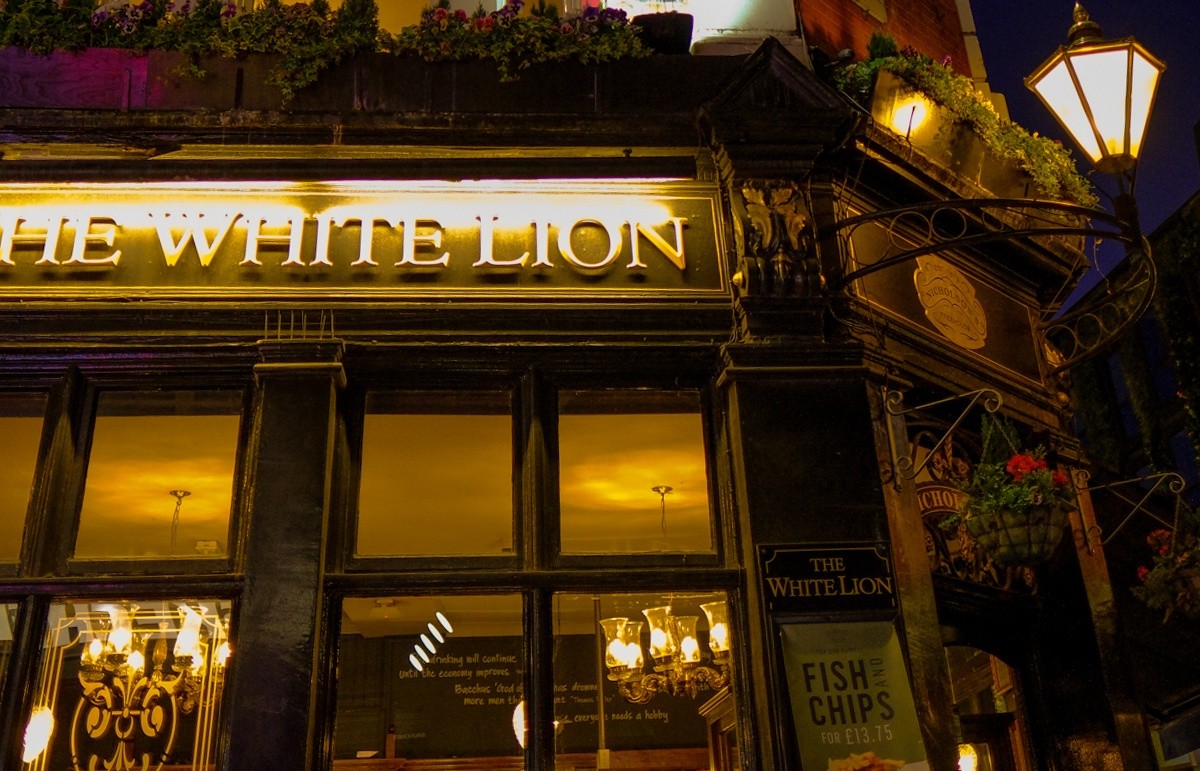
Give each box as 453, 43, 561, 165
390, 633, 736, 760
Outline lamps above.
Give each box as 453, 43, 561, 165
814, 1, 1166, 381
80, 490, 233, 714
598, 485, 731, 706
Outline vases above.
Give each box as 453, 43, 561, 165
1, 43, 751, 114
966, 506, 1069, 566
858, 68, 1085, 256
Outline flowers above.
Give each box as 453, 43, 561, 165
1131, 529, 1200, 624
838, 31, 1099, 211
937, 445, 1076, 535
0, 1, 655, 92
